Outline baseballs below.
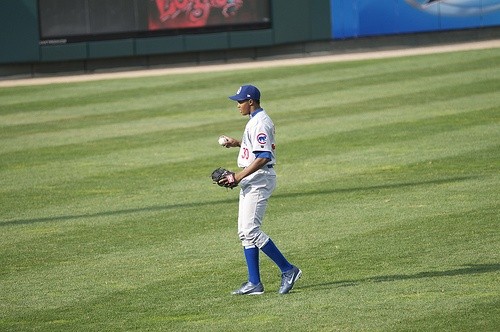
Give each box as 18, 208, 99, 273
218, 137, 227, 146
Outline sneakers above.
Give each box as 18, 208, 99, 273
231, 280, 264, 294
278, 265, 302, 294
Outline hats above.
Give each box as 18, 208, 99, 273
228, 85, 261, 102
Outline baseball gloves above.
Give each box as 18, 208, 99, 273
211, 166, 240, 191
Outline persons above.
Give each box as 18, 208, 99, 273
217, 85, 302, 296
